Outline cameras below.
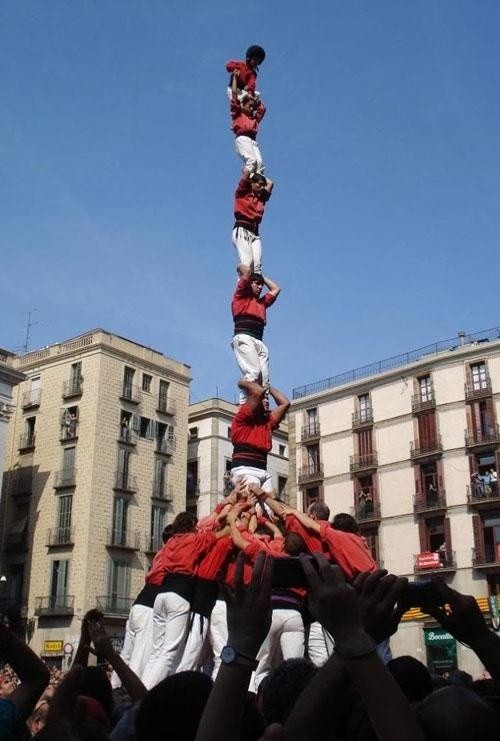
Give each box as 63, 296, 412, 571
398, 582, 432, 608
274, 557, 312, 588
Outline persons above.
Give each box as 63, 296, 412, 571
230, 261, 283, 412
230, 167, 274, 281
225, 45, 268, 111
229, 377, 292, 501
2, 468, 500, 740
229, 70, 268, 179
121, 416, 129, 442
62, 413, 77, 437
